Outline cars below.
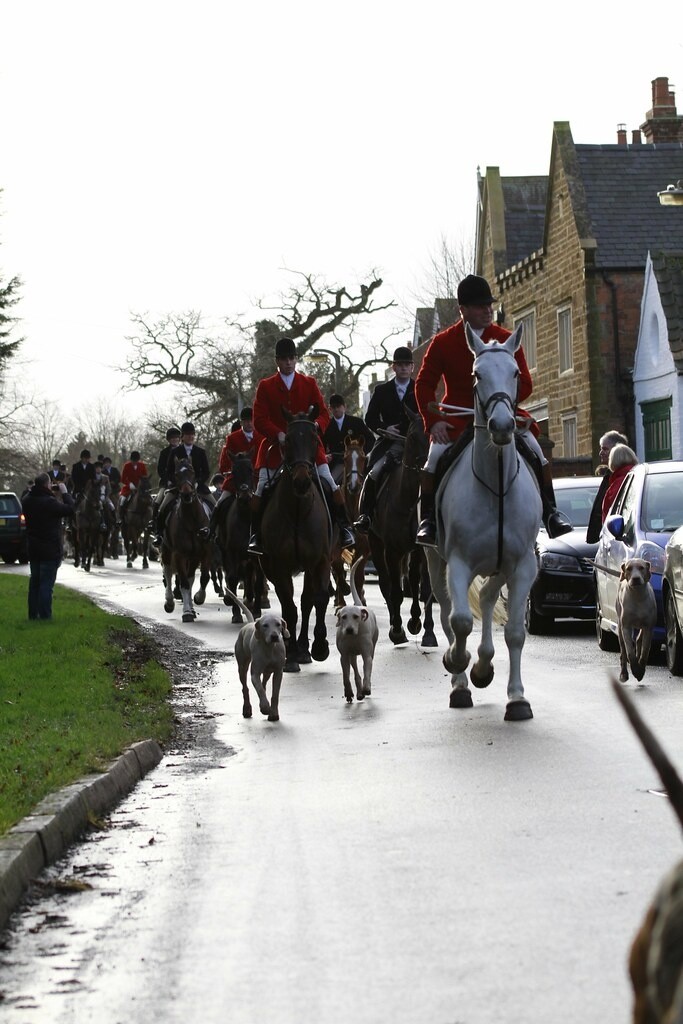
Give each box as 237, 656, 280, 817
594, 460, 683, 651
0, 492, 28, 564
524, 476, 604, 635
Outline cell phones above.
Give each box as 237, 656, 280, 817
51, 486, 59, 491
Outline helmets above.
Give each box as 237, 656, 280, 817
457, 275, 498, 305
166, 428, 180, 439
239, 408, 253, 418
53, 460, 61, 466
330, 395, 345, 407
275, 338, 297, 358
80, 450, 89, 458
182, 422, 195, 434
392, 347, 415, 364
131, 452, 139, 460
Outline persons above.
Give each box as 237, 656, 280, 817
586, 430, 628, 545
414, 275, 572, 544
148, 428, 181, 528
248, 338, 355, 549
199, 407, 267, 536
152, 423, 216, 545
22, 473, 75, 620
20, 449, 121, 529
353, 347, 441, 531
602, 443, 639, 534
117, 451, 149, 523
327, 394, 376, 485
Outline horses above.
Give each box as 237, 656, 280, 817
417, 320, 544, 721
52, 401, 439, 647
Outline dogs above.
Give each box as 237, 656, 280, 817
223, 586, 290, 721
587, 556, 657, 682
334, 555, 380, 704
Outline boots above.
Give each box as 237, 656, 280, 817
249, 492, 264, 553
203, 507, 221, 539
416, 468, 438, 545
354, 472, 377, 535
114, 504, 121, 524
333, 484, 356, 544
153, 510, 165, 546
536, 463, 572, 538
